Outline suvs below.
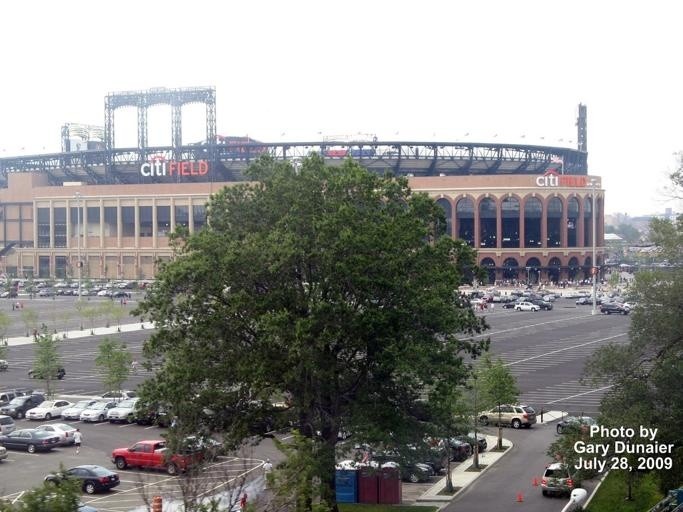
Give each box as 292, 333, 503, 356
477, 404, 537, 429
541, 463, 581, 498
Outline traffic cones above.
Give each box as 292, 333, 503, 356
532, 477, 539, 486
515, 491, 524, 502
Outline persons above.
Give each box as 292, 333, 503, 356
11, 300, 25, 310
120, 297, 129, 307
263, 458, 273, 488
132, 359, 138, 375
240, 494, 247, 512
456, 274, 633, 313
73, 428, 83, 455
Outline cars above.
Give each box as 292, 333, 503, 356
383, 445, 448, 476
0, 385, 292, 433
557, 416, 597, 435
0, 429, 60, 454
0, 360, 8, 370
34, 423, 78, 447
43, 465, 120, 494
28, 364, 66, 380
177, 437, 223, 459
454, 434, 487, 454
0, 445, 8, 461
366, 455, 434, 483
422, 437, 472, 462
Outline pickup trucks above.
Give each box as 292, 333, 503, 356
112, 440, 204, 475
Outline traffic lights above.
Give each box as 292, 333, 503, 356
589, 266, 599, 276
76, 262, 83, 268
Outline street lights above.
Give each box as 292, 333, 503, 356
587, 178, 601, 315
561, 488, 587, 512
73, 191, 83, 301
